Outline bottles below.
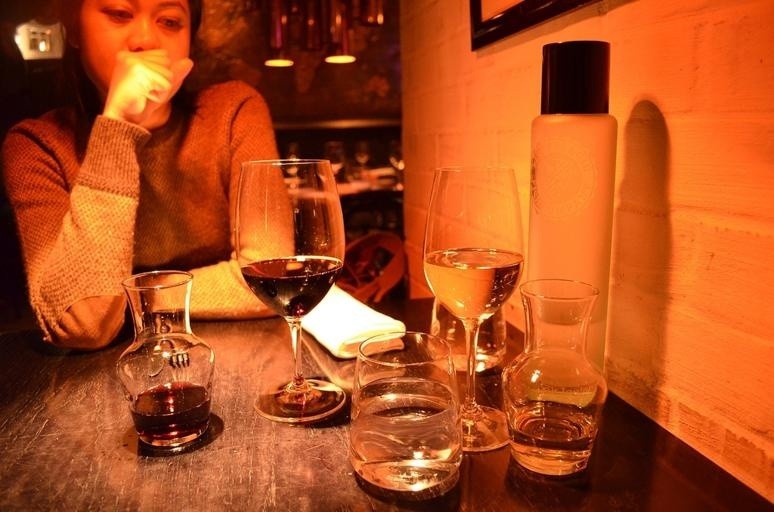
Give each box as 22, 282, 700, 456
116, 270, 218, 450
501, 277, 608, 475
527, 40, 618, 409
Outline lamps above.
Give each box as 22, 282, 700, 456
264, 0, 297, 68
322, 2, 357, 64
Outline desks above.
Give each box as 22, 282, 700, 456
0, 295, 774, 511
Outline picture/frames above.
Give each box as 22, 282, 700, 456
469, 1, 606, 52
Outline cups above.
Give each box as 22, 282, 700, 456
348, 332, 465, 491
427, 299, 507, 373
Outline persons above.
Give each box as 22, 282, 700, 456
0, 0, 297, 352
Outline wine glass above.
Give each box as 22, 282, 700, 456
234, 158, 347, 425
325, 139, 405, 190
421, 166, 526, 451
